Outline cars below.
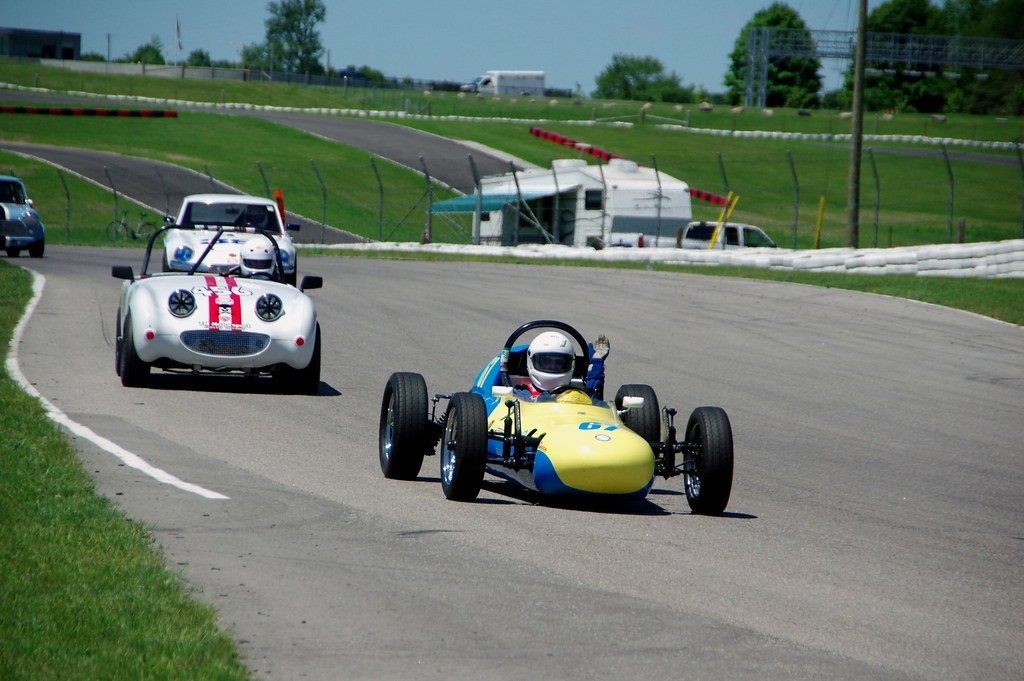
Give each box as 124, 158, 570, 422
680, 219, 777, 252
162, 190, 300, 286
1, 168, 46, 260
110, 219, 326, 391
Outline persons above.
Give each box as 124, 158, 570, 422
513, 329, 611, 409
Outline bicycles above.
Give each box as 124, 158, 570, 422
106, 209, 161, 244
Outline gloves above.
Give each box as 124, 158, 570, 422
588, 333, 610, 366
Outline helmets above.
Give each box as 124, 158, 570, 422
526, 332, 577, 392
238, 239, 276, 280
